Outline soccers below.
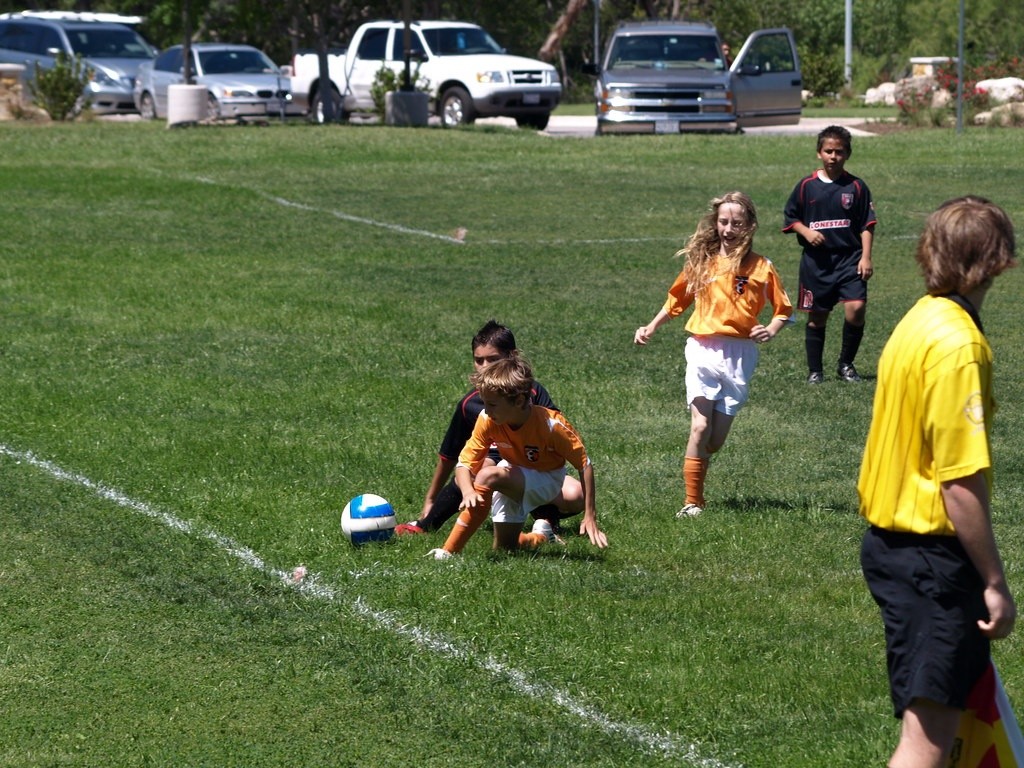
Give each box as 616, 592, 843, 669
339, 492, 397, 545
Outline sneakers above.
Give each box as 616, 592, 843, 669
807, 371, 824, 385
395, 520, 426, 537
531, 520, 556, 545
676, 503, 703, 518
837, 361, 861, 383
422, 547, 452, 560
528, 506, 561, 534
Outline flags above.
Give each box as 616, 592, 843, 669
945, 652, 1024, 768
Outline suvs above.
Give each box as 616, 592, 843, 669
581, 18, 804, 138
0, 11, 156, 114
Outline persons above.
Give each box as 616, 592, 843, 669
393, 318, 585, 545
782, 126, 878, 385
854, 193, 1018, 768
698, 42, 745, 134
422, 350, 608, 561
634, 191, 794, 517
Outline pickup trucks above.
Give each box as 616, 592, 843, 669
289, 20, 563, 133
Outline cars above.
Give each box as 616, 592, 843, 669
132, 41, 293, 123
